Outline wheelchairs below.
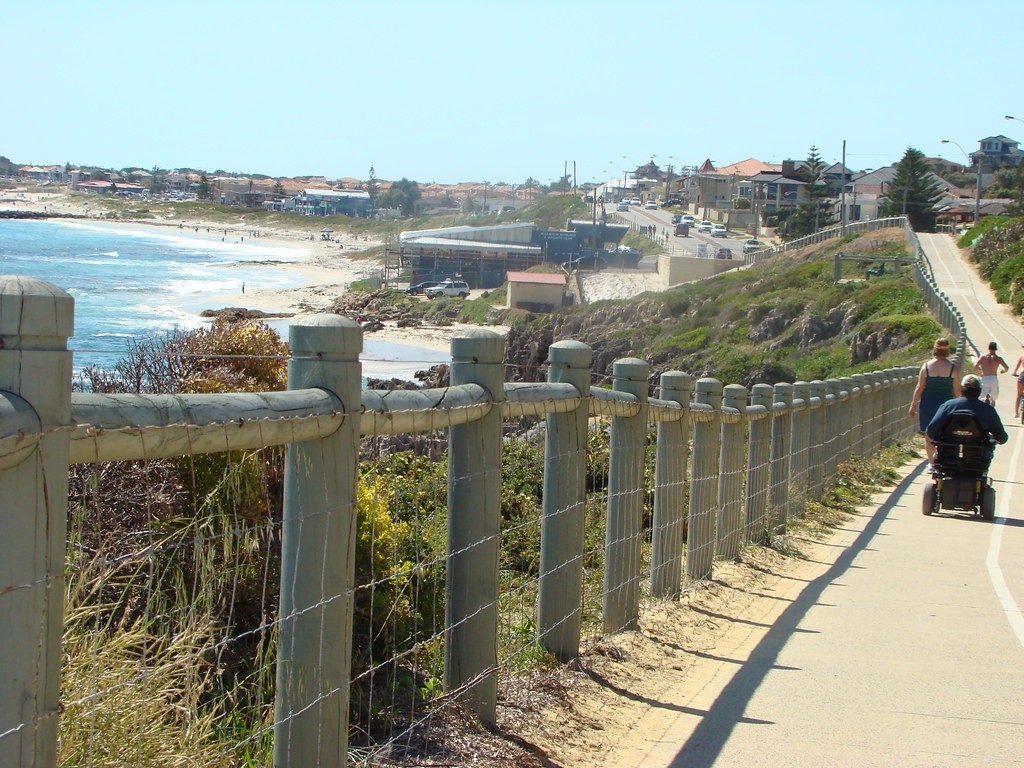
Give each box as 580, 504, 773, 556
920, 410, 997, 518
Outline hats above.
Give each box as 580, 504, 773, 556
961, 373, 983, 389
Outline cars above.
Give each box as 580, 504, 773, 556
405, 280, 439, 296
698, 220, 714, 234
711, 223, 728, 238
621, 196, 631, 205
585, 196, 594, 204
616, 202, 629, 211
630, 197, 642, 206
672, 215, 682, 224
661, 198, 682, 208
714, 247, 733, 261
562, 254, 607, 272
744, 239, 760, 253
645, 201, 657, 210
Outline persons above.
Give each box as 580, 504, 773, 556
926, 374, 1009, 445
974, 342, 1009, 407
909, 337, 960, 474
648, 225, 669, 243
196, 226, 343, 294
1012, 345, 1024, 418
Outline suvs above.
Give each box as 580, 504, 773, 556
674, 223, 690, 238
425, 279, 471, 301
681, 215, 696, 227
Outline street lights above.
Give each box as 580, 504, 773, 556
784, 189, 820, 233
942, 139, 981, 231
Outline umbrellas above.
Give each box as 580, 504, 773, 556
321, 228, 334, 240
